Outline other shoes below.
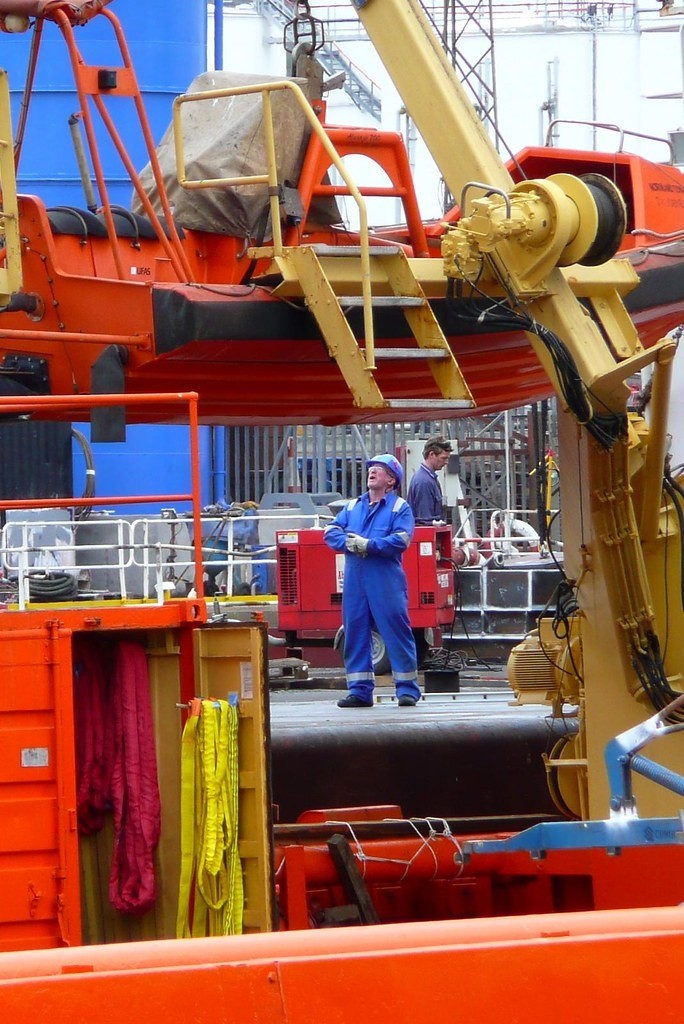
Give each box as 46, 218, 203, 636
337, 694, 370, 707
399, 695, 415, 706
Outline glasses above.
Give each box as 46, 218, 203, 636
368, 467, 394, 478
429, 441, 451, 448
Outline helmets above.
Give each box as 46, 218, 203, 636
365, 454, 402, 486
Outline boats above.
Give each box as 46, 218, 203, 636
0, 2, 684, 432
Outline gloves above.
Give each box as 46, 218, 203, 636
346, 533, 369, 552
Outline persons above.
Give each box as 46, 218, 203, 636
323, 434, 453, 708
